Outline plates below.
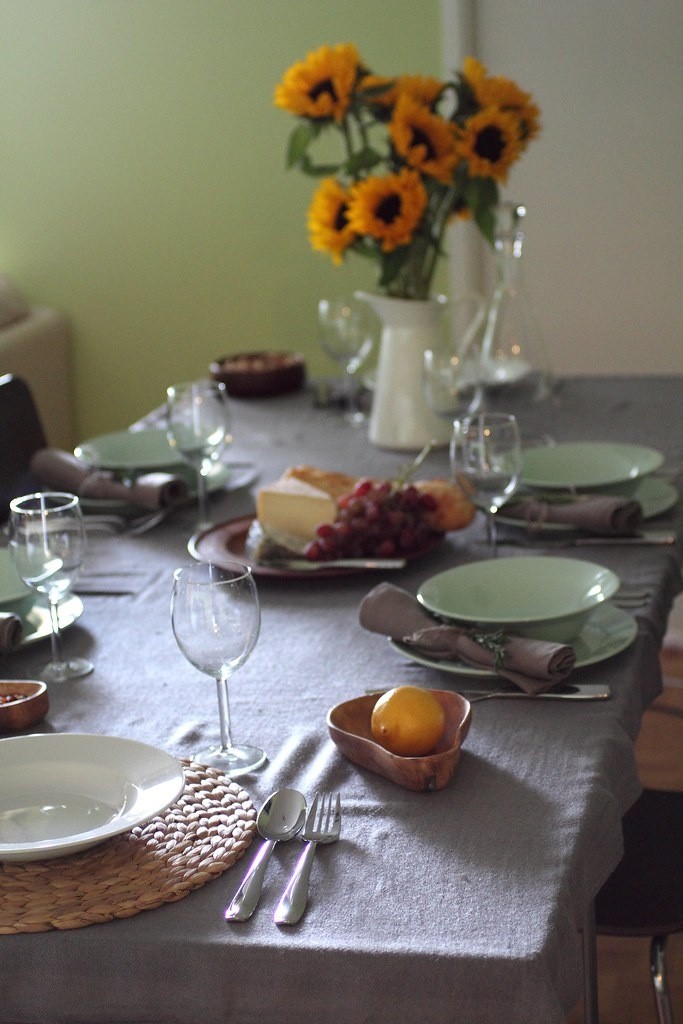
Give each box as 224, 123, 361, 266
188, 461, 229, 498
494, 478, 678, 530
0, 733, 186, 861
364, 360, 531, 394
0, 592, 84, 647
388, 606, 641, 676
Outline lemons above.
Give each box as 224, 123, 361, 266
371, 686, 447, 760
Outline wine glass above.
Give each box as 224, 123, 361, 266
171, 560, 267, 776
449, 412, 521, 559
9, 491, 93, 682
320, 298, 374, 425
167, 378, 236, 540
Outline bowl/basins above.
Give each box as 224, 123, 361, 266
0, 546, 54, 621
73, 428, 219, 488
326, 687, 472, 791
494, 444, 666, 498
210, 352, 305, 398
0, 679, 49, 733
416, 556, 621, 644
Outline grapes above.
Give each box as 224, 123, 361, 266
288, 430, 447, 570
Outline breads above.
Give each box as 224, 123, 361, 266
260, 474, 336, 545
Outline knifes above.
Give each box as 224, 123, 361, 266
471, 538, 677, 545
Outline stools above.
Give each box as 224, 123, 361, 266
570, 789, 682, 1024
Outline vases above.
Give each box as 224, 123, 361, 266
351, 290, 464, 451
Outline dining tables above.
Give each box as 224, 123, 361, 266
0, 363, 682, 1024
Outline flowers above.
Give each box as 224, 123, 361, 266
268, 36, 546, 302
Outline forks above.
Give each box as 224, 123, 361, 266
273, 791, 343, 926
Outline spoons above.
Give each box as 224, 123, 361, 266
225, 789, 307, 921
17, 508, 170, 537
